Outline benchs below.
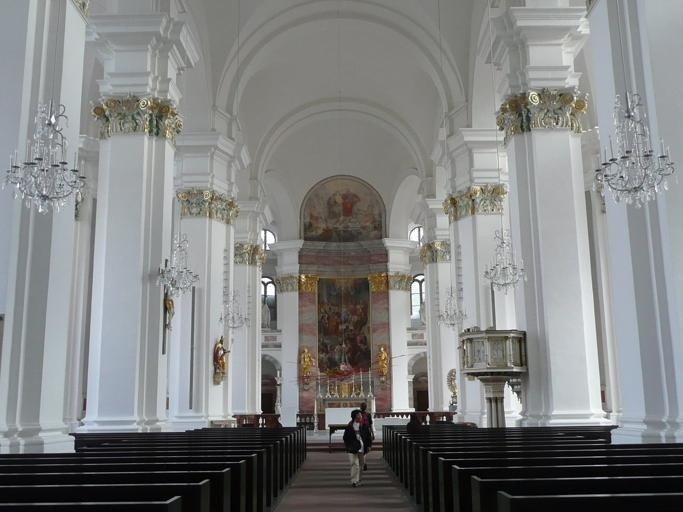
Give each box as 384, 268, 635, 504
0, 426, 307, 512
382, 425, 682, 512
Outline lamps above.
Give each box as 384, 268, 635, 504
217, 0, 251, 329
1, 0, 89, 216
435, 0, 469, 331
482, 1, 529, 297
592, 0, 679, 209
159, 1, 200, 296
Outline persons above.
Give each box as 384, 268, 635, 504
213, 335, 231, 376
446, 369, 457, 395
298, 346, 314, 376
358, 402, 374, 471
342, 409, 371, 487
375, 345, 389, 375
317, 298, 370, 374
307, 188, 377, 242
163, 290, 175, 329
